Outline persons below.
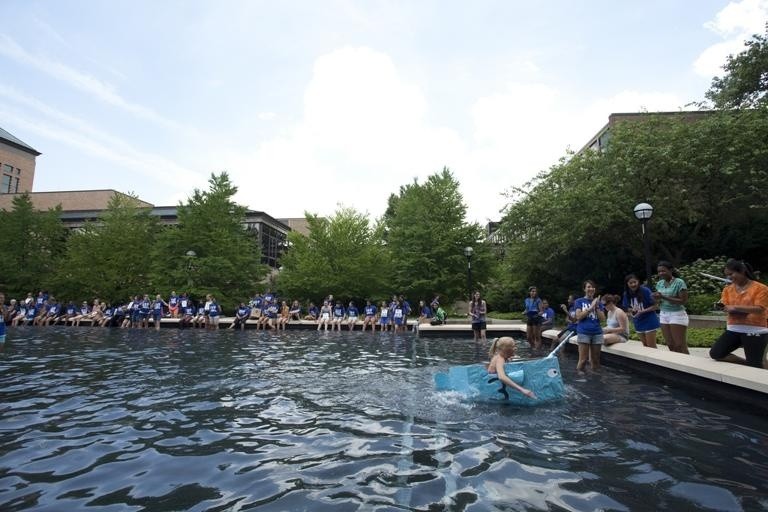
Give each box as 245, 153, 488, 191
599, 295, 629, 345
1, 292, 9, 347
621, 274, 659, 349
229, 286, 445, 335
576, 281, 606, 370
488, 336, 537, 399
468, 290, 487, 342
548, 294, 579, 357
522, 285, 544, 353
539, 298, 554, 332
652, 261, 689, 352
7, 291, 221, 330
709, 261, 768, 368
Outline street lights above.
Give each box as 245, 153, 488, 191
462, 244, 473, 302
186, 250, 197, 269
632, 198, 655, 294
277, 239, 294, 272
